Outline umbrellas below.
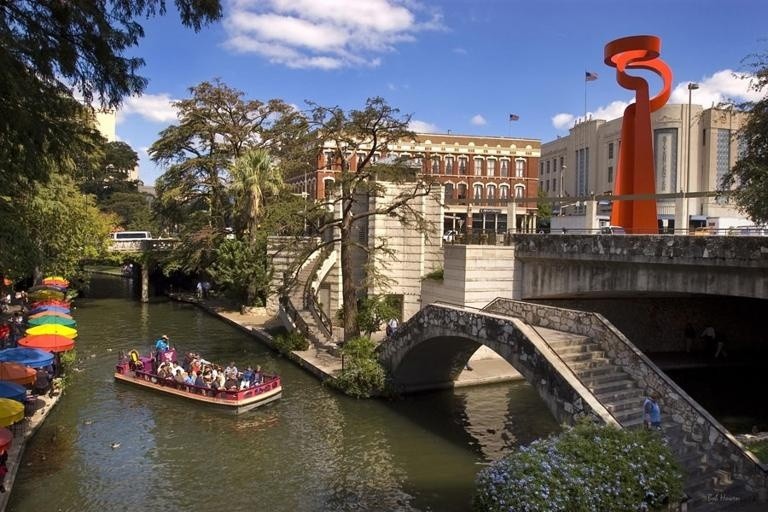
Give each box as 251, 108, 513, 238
0, 276, 78, 455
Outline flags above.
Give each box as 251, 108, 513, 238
510, 114, 519, 120
586, 72, 598, 81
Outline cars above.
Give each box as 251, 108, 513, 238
693, 218, 761, 237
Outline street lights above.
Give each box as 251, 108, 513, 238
684, 82, 700, 235
558, 163, 567, 217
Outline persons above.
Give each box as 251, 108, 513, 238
685, 319, 737, 362
155, 335, 264, 396
642, 391, 661, 430
388, 314, 399, 336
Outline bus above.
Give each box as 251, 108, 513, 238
106, 231, 152, 251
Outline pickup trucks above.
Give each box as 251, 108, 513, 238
442, 230, 464, 244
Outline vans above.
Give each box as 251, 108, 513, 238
600, 225, 625, 235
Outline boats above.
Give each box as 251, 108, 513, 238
112, 348, 282, 418
113, 385, 278, 434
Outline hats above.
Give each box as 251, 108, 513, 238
162, 335, 169, 340
205, 367, 211, 373
